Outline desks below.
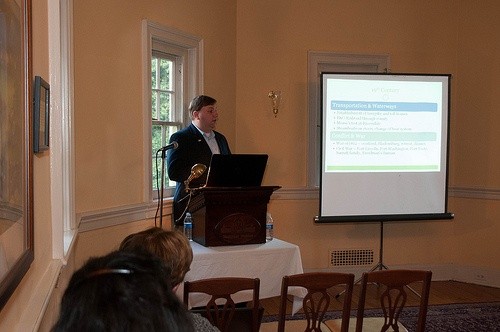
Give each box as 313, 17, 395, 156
185, 237, 309, 317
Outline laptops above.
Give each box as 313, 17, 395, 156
203, 153, 269, 186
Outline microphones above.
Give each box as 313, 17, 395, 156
158, 141, 179, 152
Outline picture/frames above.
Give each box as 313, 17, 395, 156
32, 76, 50, 154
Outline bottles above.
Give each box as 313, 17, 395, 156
184, 213, 193, 241
266, 212, 274, 240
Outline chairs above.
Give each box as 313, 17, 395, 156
183, 269, 433, 332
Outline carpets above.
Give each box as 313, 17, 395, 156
260, 300, 500, 332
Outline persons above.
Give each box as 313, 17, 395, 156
50, 227, 219, 332
167, 95, 232, 234
